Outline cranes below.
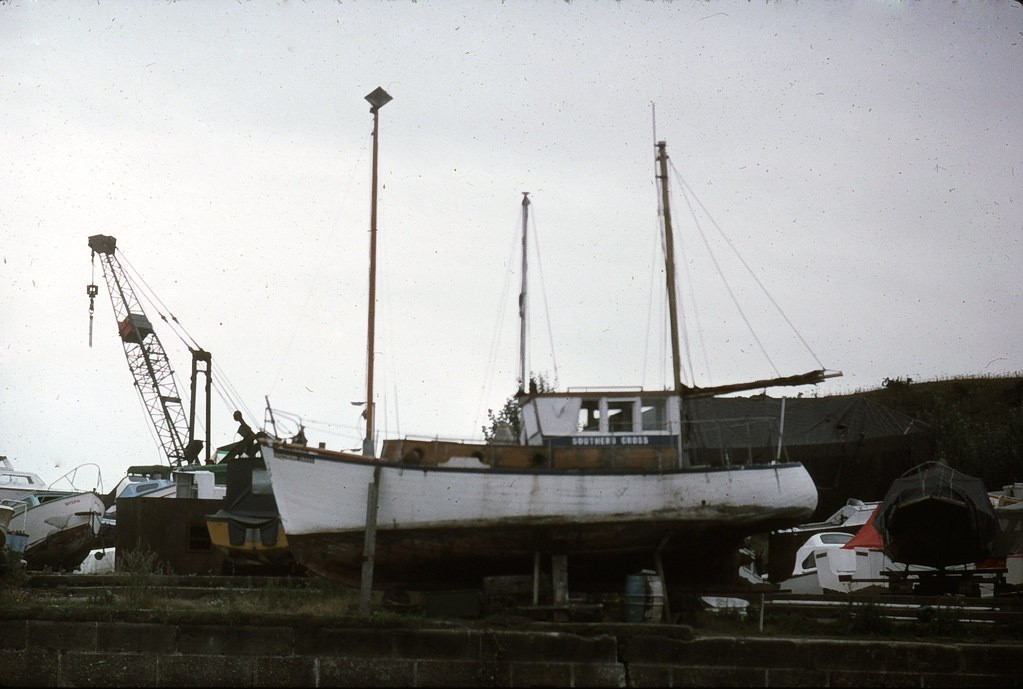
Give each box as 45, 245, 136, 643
85, 232, 214, 472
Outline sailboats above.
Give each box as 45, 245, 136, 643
253, 95, 820, 596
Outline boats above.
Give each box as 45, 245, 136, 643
706, 457, 1023, 644
0, 396, 325, 584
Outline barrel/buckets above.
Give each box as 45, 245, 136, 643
622, 574, 646, 623
643, 574, 665, 623
